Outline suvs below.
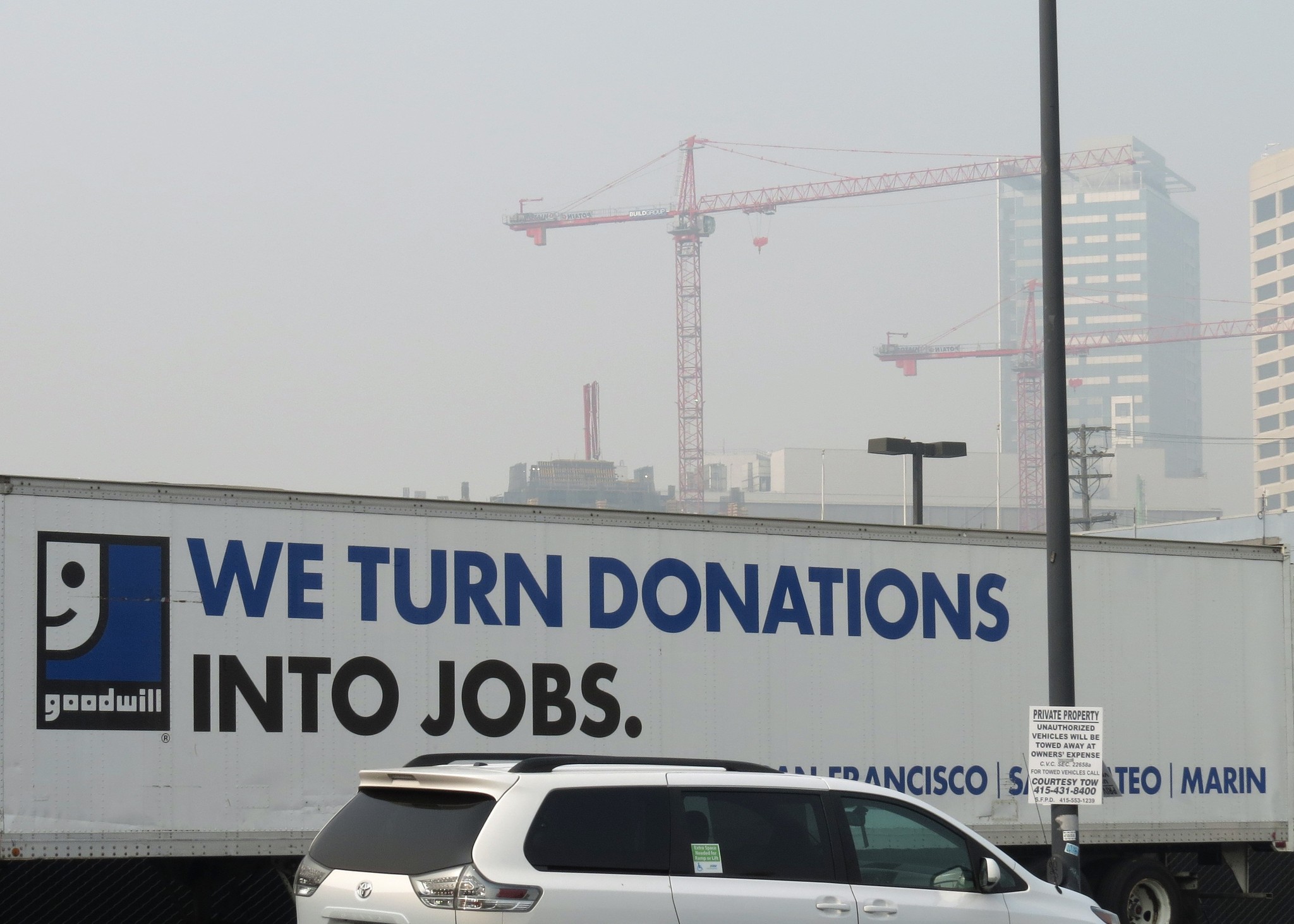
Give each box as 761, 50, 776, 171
291, 751, 1124, 924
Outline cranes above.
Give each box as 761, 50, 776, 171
875, 277, 1294, 534
504, 133, 1144, 511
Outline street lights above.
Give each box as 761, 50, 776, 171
867, 438, 967, 528
